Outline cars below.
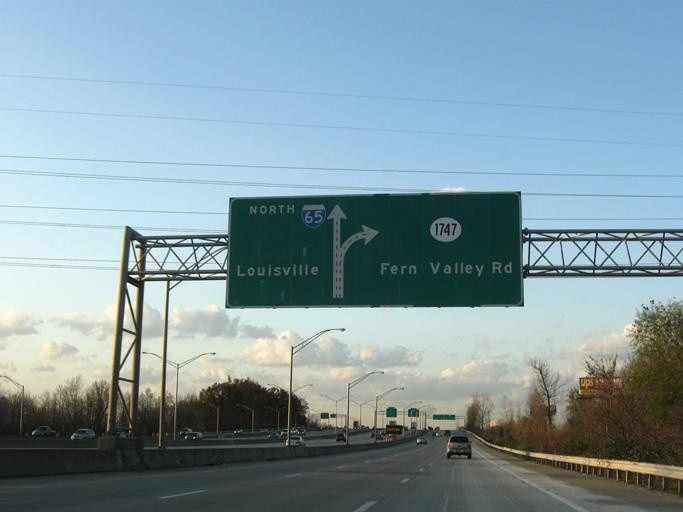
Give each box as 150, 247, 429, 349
266, 426, 306, 447
179, 427, 204, 441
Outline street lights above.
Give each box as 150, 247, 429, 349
319, 371, 438, 446
286, 327, 346, 448
201, 399, 280, 435
142, 351, 217, 439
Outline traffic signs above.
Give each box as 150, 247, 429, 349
223, 190, 525, 310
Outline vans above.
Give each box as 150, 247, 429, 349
70, 428, 96, 441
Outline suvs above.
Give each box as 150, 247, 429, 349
445, 435, 473, 459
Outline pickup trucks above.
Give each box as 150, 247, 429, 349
31, 425, 56, 436
